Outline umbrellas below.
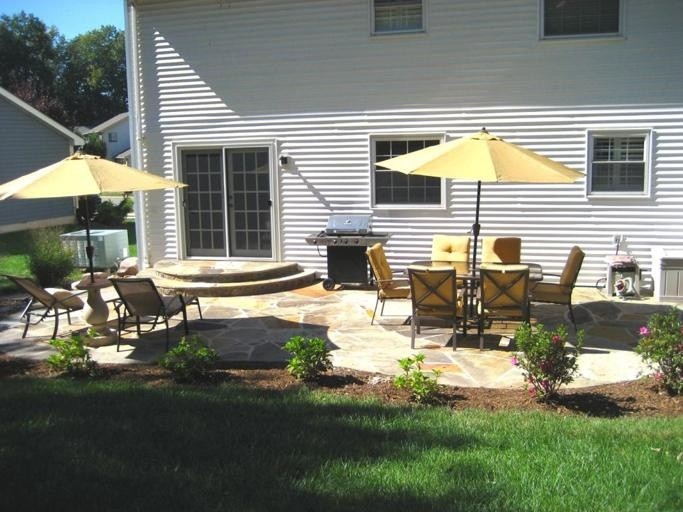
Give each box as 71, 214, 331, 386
370, 126, 587, 317
0, 144, 189, 281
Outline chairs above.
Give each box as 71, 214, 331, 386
365, 234, 585, 349
0, 274, 202, 354
